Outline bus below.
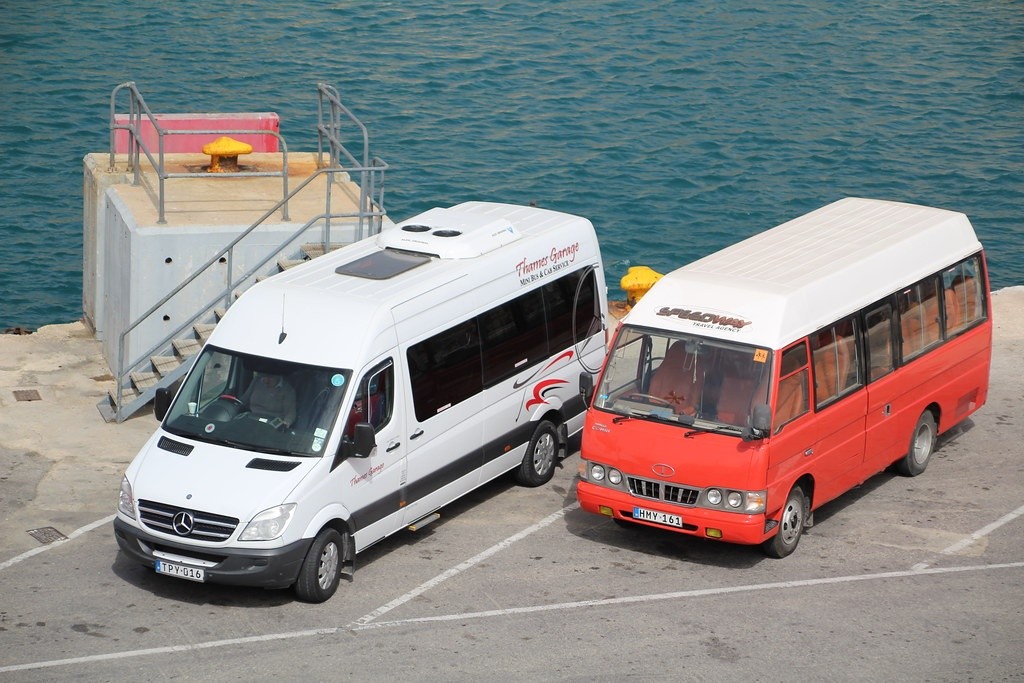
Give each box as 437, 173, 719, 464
576, 196, 994, 559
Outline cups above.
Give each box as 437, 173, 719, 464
187, 402, 197, 415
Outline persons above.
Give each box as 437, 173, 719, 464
241, 371, 297, 427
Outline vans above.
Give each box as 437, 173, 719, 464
112, 200, 610, 604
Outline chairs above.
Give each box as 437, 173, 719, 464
648, 339, 713, 416
952, 274, 980, 326
715, 354, 758, 426
752, 353, 805, 432
926, 289, 958, 343
812, 334, 852, 400
898, 303, 925, 359
867, 315, 893, 381
